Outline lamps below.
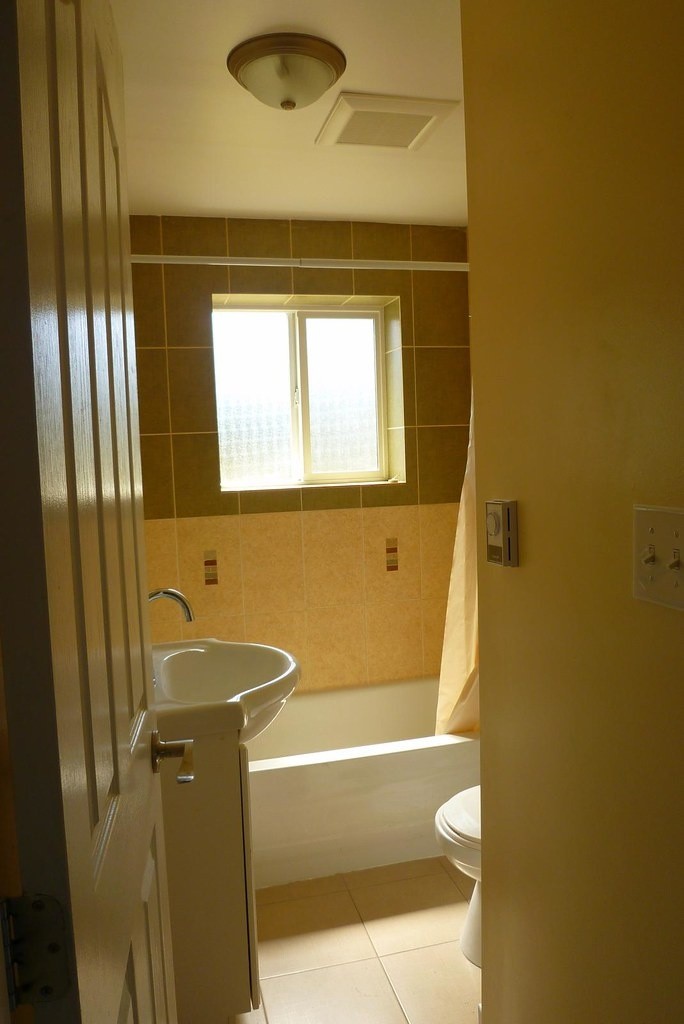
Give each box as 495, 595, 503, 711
226, 32, 346, 110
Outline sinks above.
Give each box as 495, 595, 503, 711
153, 638, 299, 745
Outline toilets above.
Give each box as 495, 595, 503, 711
434, 783, 484, 971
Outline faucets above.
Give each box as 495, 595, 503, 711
147, 588, 195, 621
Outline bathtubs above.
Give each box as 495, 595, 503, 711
240, 673, 481, 891
